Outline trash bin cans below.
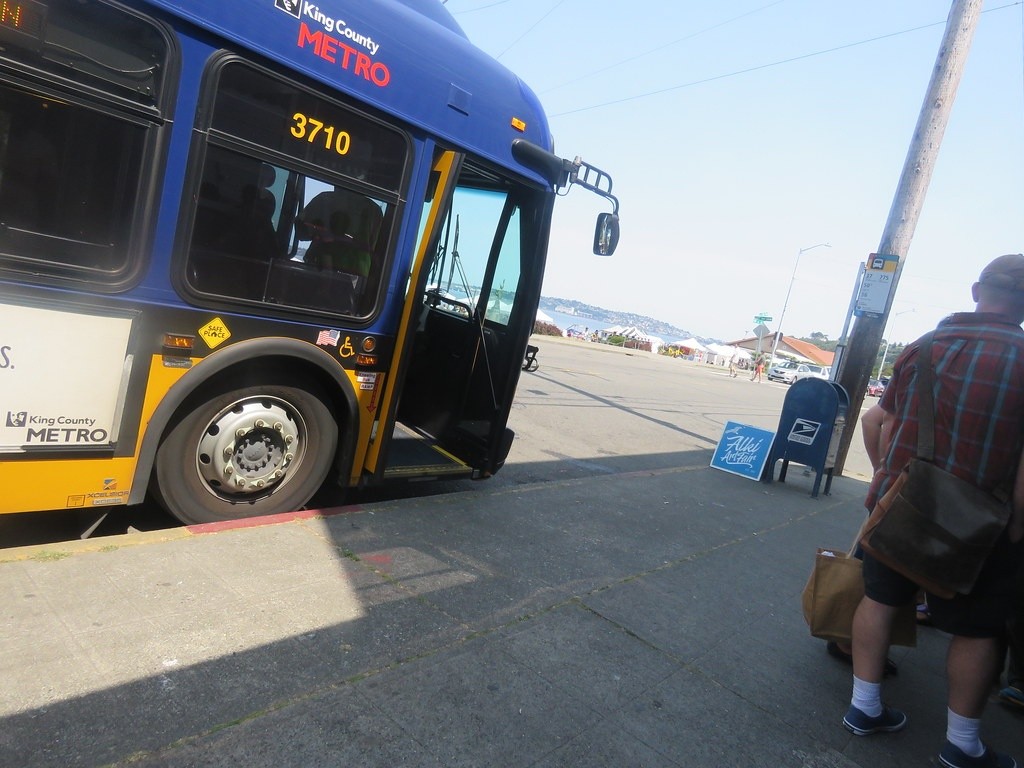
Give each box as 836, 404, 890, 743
765, 377, 850, 498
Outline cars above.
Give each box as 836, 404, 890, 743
767, 362, 885, 397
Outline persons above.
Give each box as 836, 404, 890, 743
567, 327, 599, 342
750, 351, 766, 383
826, 253, 1024, 768
728, 343, 740, 377
295, 159, 383, 297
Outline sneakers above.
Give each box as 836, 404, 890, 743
843, 699, 907, 736
939, 736, 1017, 768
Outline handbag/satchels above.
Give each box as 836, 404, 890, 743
800, 512, 918, 648
857, 458, 1011, 603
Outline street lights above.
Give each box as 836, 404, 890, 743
767, 243, 833, 375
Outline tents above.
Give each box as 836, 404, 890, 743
601, 324, 751, 366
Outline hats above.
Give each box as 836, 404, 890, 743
979, 253, 1024, 293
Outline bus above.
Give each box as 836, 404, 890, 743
1, 1, 619, 525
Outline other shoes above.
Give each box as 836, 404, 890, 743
916, 611, 936, 626
999, 686, 1024, 707
841, 652, 897, 673
